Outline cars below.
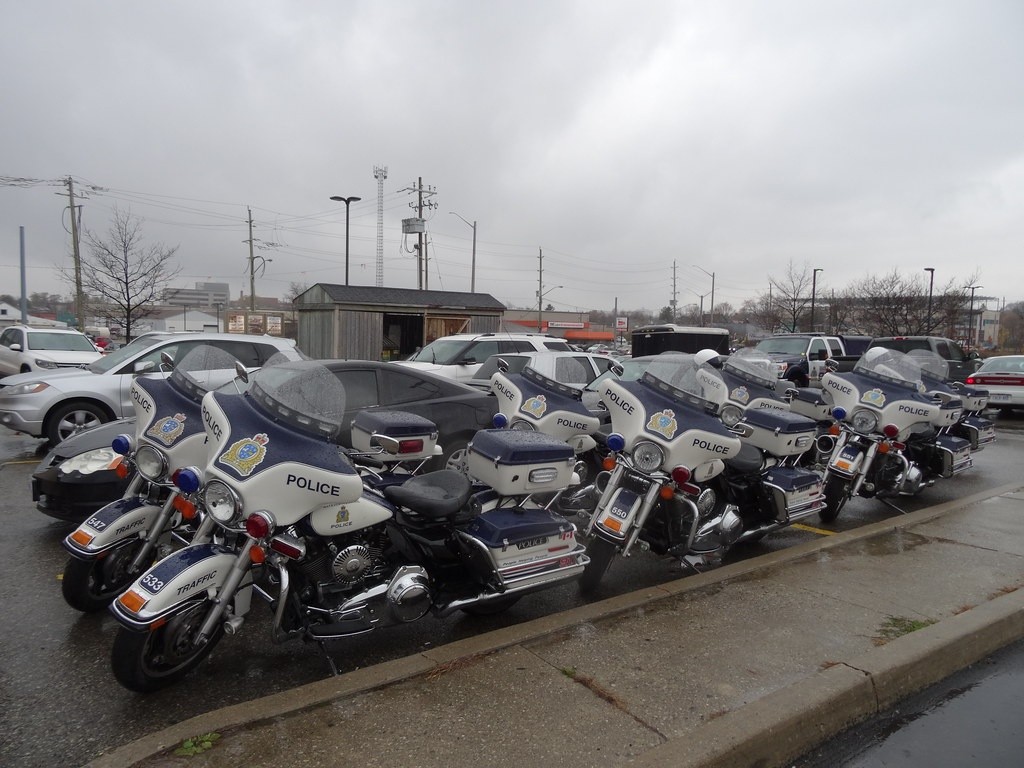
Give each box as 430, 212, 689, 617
0, 323, 1024, 526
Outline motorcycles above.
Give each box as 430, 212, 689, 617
816, 345, 973, 524
56, 342, 442, 617
898, 348, 996, 481
106, 358, 590, 695
578, 351, 827, 593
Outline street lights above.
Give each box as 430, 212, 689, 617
924, 267, 935, 335
692, 263, 715, 328
810, 269, 823, 331
251, 258, 274, 310
538, 284, 565, 332
448, 211, 478, 292
328, 194, 361, 286
962, 285, 984, 348
